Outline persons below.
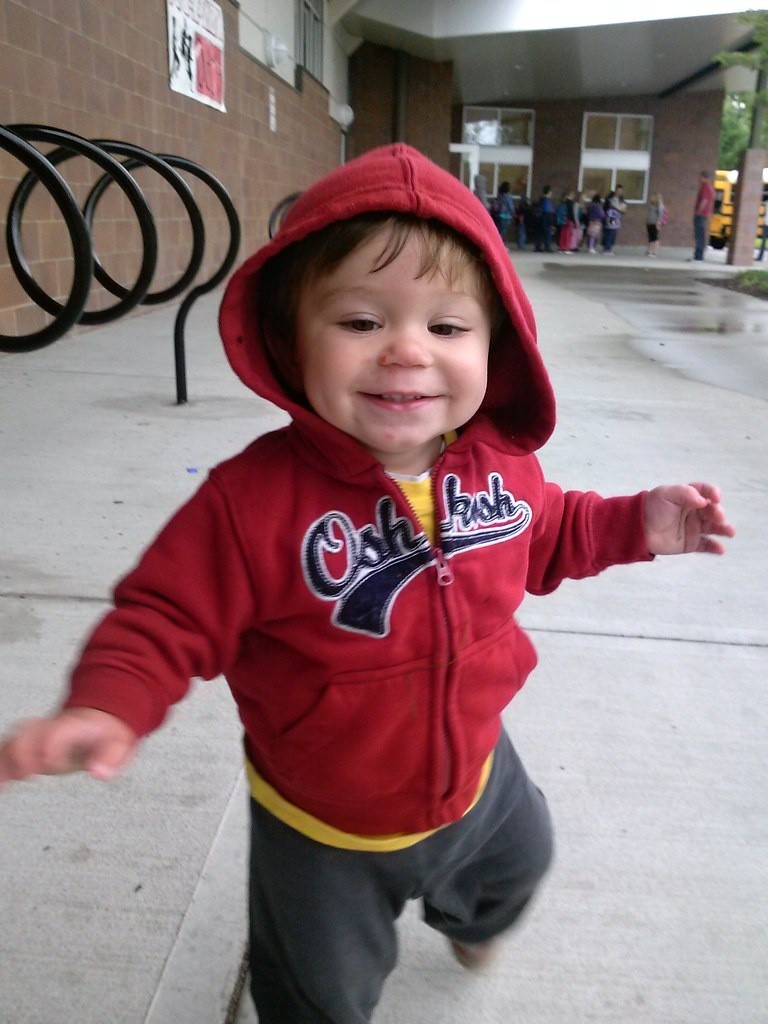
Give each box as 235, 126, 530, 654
1, 141, 736, 1023
494, 181, 627, 253
646, 191, 664, 260
690, 168, 714, 263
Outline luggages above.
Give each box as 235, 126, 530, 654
557, 221, 581, 252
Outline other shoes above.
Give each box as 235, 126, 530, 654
533, 248, 543, 252
586, 245, 598, 254
602, 250, 617, 256
448, 939, 500, 974
545, 249, 554, 253
647, 251, 656, 257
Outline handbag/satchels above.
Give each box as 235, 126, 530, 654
499, 206, 516, 221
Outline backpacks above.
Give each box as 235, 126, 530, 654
659, 208, 669, 227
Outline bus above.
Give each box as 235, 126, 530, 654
708, 168, 766, 251
